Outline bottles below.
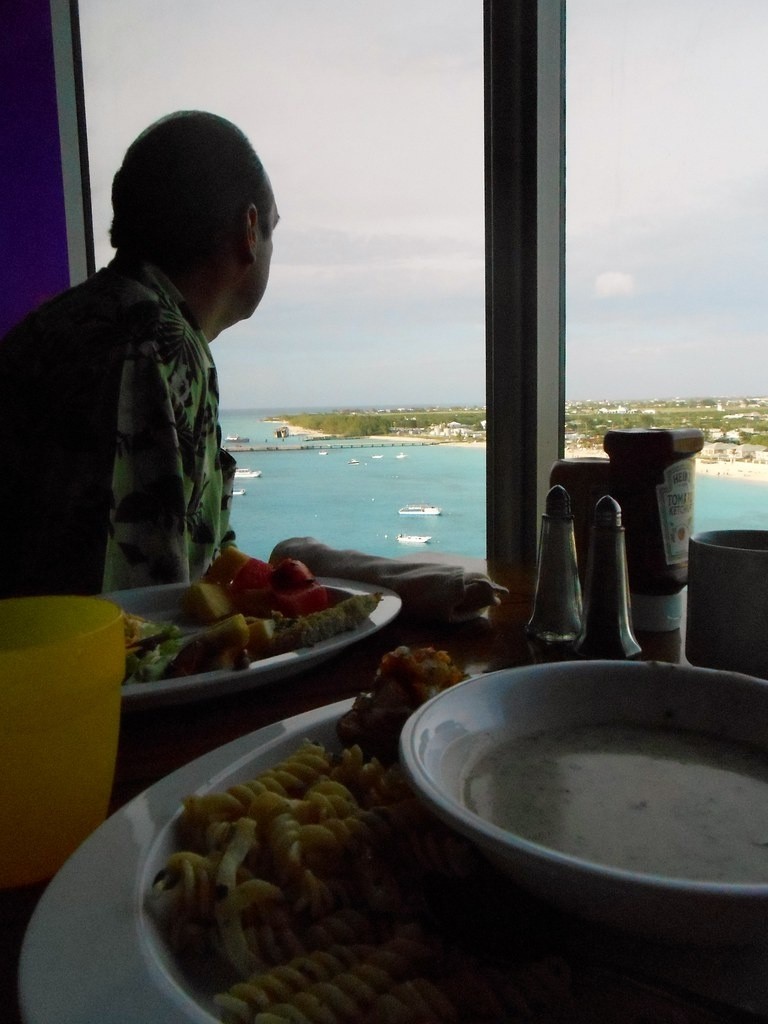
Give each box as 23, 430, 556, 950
547, 455, 611, 596
524, 483, 584, 643
568, 492, 642, 659
604, 427, 703, 631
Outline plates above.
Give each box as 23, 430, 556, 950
99, 573, 402, 698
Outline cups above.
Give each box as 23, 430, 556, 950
684, 527, 768, 678
0, 597, 127, 888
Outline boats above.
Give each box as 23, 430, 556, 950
235, 468, 262, 477
348, 459, 359, 465
319, 449, 328, 455
233, 489, 247, 494
396, 529, 432, 543
398, 504, 442, 514
224, 433, 249, 443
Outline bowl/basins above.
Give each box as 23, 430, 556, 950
399, 658, 768, 947
15, 698, 379, 1023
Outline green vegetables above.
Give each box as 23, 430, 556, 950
126, 614, 186, 685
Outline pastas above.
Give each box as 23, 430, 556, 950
159, 736, 574, 1024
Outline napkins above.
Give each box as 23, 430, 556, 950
268, 535, 510, 625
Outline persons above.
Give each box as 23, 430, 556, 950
0, 110, 281, 600
